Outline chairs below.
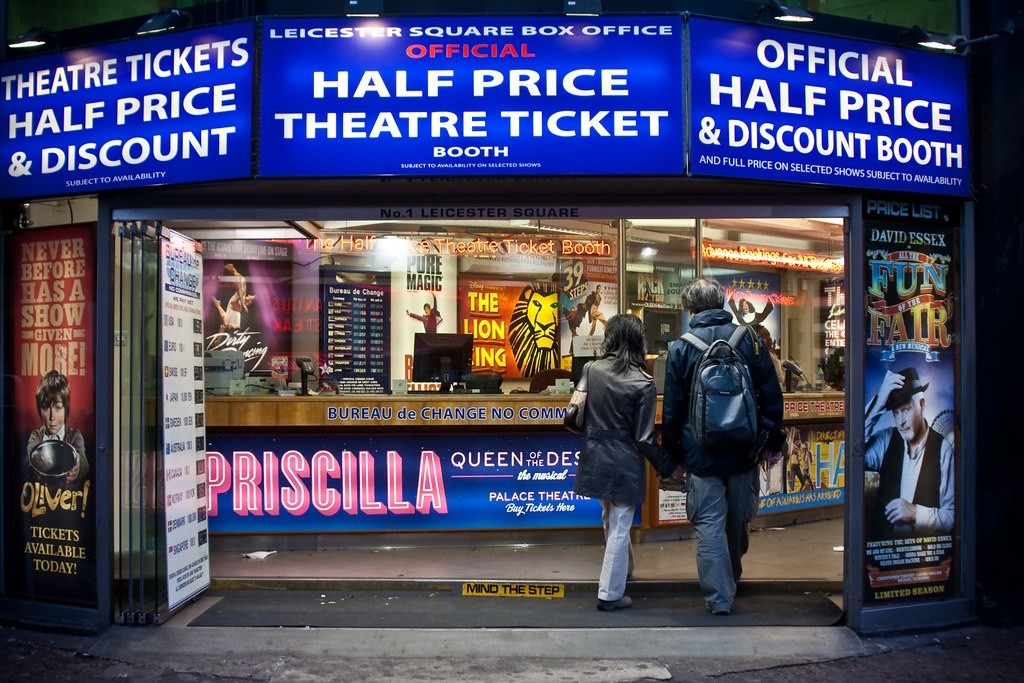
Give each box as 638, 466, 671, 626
530, 369, 576, 393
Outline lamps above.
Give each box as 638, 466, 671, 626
9, 26, 61, 51
897, 24, 956, 50
755, 1, 814, 23
562, 0, 602, 16
345, 0, 384, 18
511, 219, 669, 245
136, 9, 193, 35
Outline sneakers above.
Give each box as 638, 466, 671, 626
597, 597, 632, 611
626, 575, 632, 580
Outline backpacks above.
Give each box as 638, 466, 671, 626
679, 325, 758, 450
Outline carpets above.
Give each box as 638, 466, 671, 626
187, 590, 844, 628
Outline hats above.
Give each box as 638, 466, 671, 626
885, 368, 929, 411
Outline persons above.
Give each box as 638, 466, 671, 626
865, 367, 955, 534
727, 292, 778, 345
564, 314, 687, 612
563, 286, 608, 353
406, 291, 437, 335
211, 265, 256, 347
662, 279, 787, 616
24, 370, 90, 485
787, 424, 815, 492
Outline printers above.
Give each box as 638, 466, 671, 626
244, 370, 288, 395
203, 350, 245, 396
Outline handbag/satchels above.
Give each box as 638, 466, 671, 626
564, 361, 593, 435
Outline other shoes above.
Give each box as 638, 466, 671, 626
706, 603, 731, 615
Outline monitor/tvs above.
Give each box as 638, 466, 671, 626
644, 307, 682, 355
412, 333, 474, 394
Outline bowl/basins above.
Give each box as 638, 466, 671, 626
29, 441, 77, 480
